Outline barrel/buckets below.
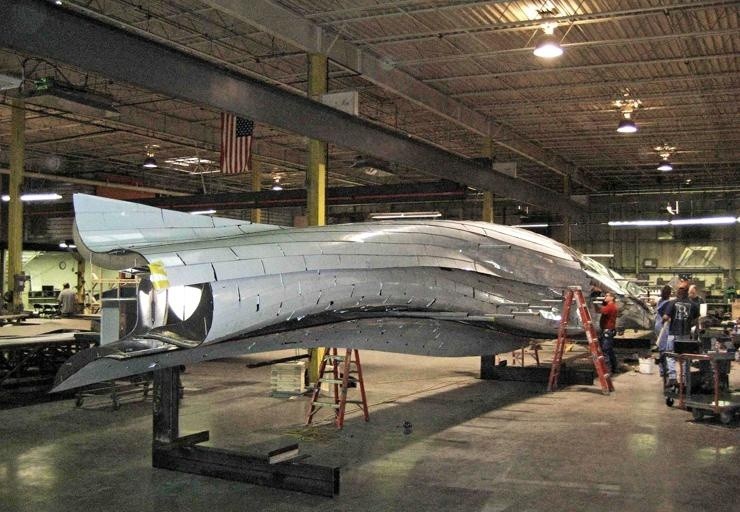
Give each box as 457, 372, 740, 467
639, 358, 655, 377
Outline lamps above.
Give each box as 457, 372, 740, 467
23, 78, 120, 118
143, 146, 158, 168
616, 106, 637, 133
350, 158, 396, 179
656, 151, 672, 173
271, 178, 283, 191
2, 193, 63, 201
533, 22, 563, 58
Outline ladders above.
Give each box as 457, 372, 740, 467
304, 346, 371, 431
512, 344, 539, 369
547, 286, 614, 396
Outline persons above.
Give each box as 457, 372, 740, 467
595, 291, 619, 375
654, 281, 705, 388
56, 283, 78, 317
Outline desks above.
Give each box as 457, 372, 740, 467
0, 331, 101, 397
